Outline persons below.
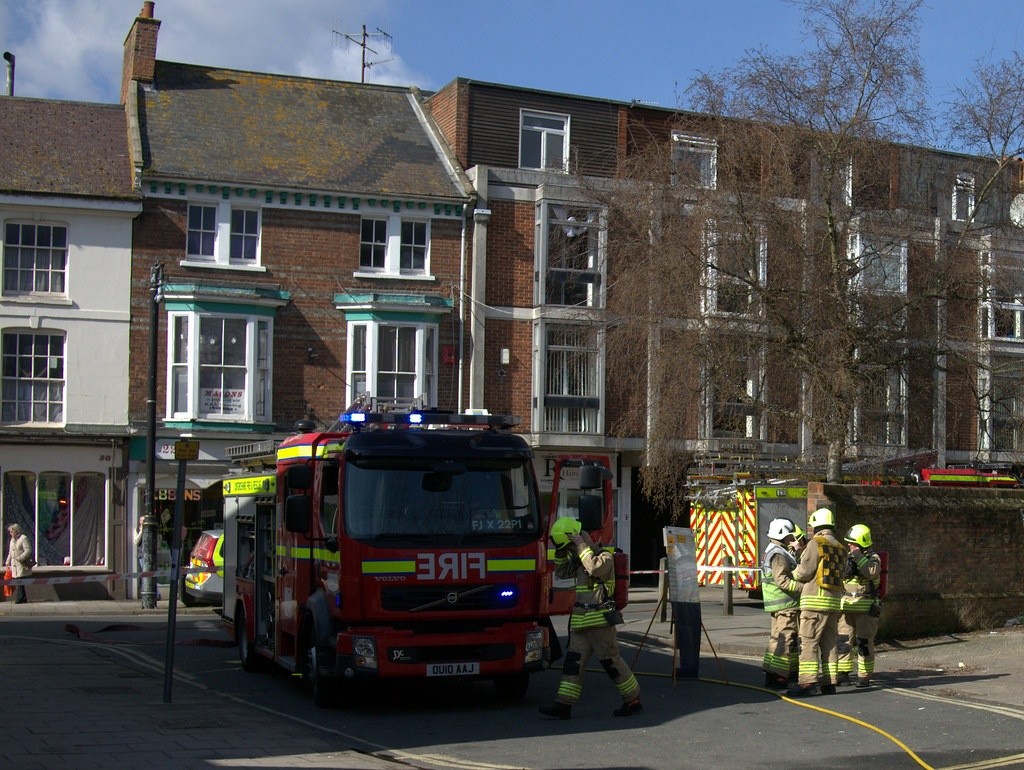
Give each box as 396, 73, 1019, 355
5, 523, 32, 604
133, 516, 161, 601
538, 518, 644, 719
761, 508, 881, 696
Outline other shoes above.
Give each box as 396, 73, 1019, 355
780, 674, 798, 689
15, 598, 27, 603
764, 671, 777, 689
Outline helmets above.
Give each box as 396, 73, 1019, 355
550, 516, 582, 550
807, 508, 835, 528
843, 524, 873, 548
793, 524, 804, 541
766, 518, 796, 540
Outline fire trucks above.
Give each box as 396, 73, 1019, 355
220, 391, 613, 708
683, 449, 1024, 599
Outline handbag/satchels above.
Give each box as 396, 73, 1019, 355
4, 566, 16, 596
21, 554, 36, 569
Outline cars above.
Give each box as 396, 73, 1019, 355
186, 530, 224, 606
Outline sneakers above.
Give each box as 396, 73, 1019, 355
856, 676, 869, 687
835, 673, 850, 686
540, 701, 572, 721
613, 700, 643, 717
820, 685, 837, 695
787, 680, 819, 698
